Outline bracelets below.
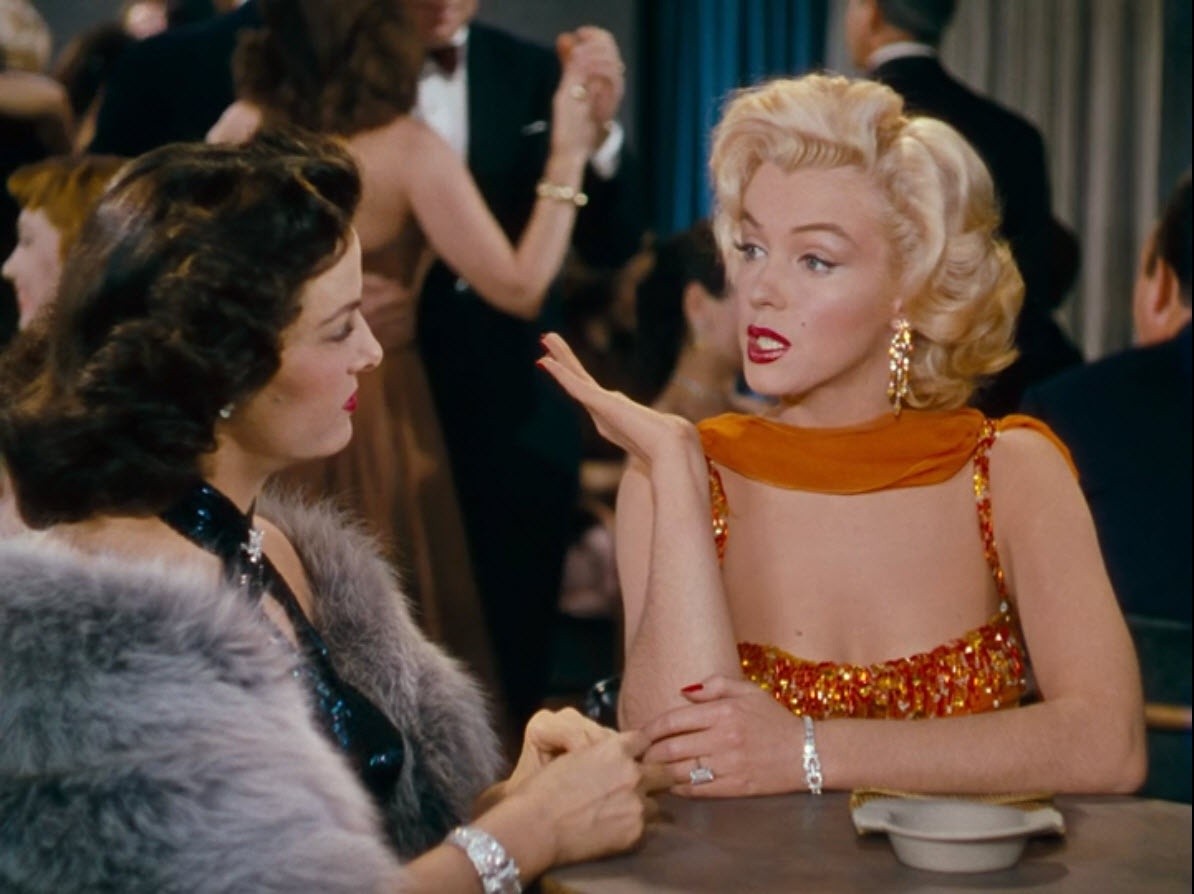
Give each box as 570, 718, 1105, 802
445, 825, 523, 894
803, 716, 823, 798
537, 182, 588, 206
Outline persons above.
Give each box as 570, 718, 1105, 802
538, 70, 1147, 798
0, 0, 1194, 755
0, 132, 651, 894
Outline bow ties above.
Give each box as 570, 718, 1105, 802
423, 44, 460, 76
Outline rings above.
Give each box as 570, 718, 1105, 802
689, 756, 714, 784
569, 85, 589, 101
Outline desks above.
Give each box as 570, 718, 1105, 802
539, 782, 1193, 894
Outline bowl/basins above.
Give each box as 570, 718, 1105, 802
855, 799, 1063, 872
